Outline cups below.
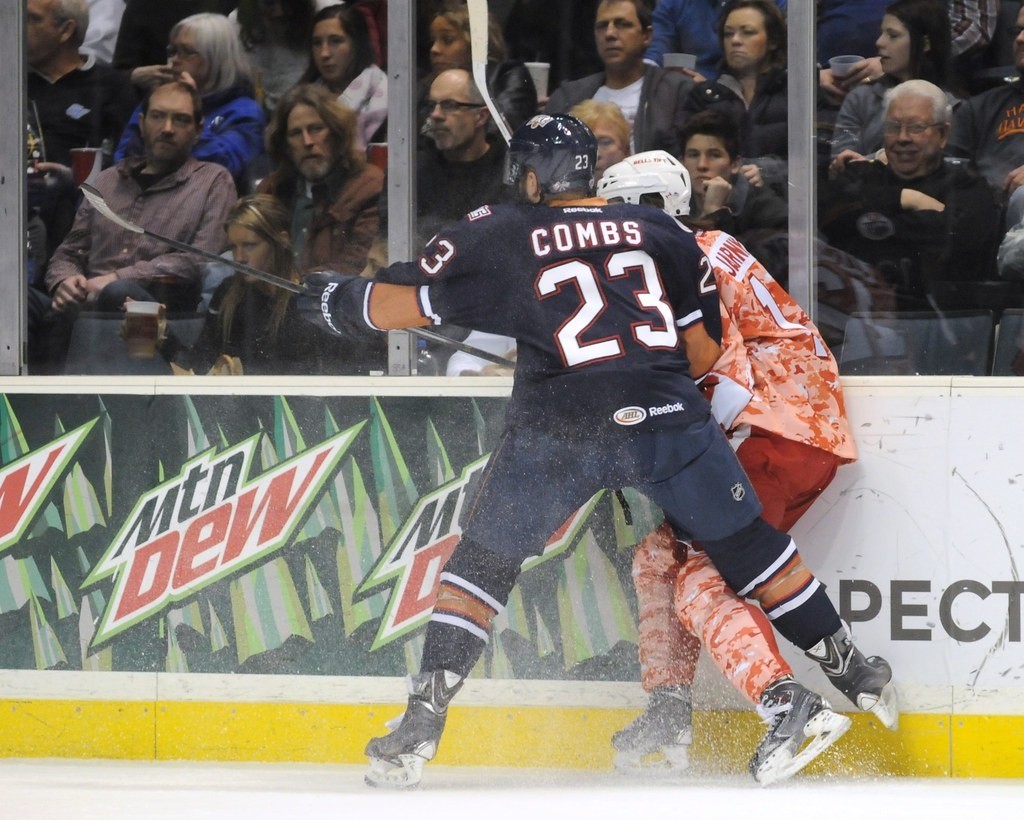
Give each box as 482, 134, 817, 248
126, 302, 159, 357
71, 148, 99, 184
828, 56, 865, 90
368, 143, 387, 170
524, 62, 550, 100
662, 53, 696, 69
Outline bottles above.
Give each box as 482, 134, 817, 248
417, 340, 437, 376
26, 123, 39, 174
27, 231, 35, 282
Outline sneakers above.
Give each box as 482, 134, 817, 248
747, 673, 852, 786
611, 684, 694, 784
362, 670, 465, 791
805, 625, 900, 730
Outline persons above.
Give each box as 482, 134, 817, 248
296, 113, 894, 791
677, 111, 789, 232
25, 0, 1024, 373
113, 13, 267, 181
594, 150, 857, 782
824, 81, 998, 314
119, 194, 345, 373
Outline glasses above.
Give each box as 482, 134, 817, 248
423, 98, 486, 112
881, 119, 947, 134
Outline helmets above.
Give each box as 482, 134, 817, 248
503, 113, 598, 194
593, 148, 691, 217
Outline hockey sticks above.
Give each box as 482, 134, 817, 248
465, 1, 516, 147
78, 179, 518, 374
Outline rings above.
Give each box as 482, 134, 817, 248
865, 77, 870, 83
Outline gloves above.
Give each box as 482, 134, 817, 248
297, 269, 387, 344
